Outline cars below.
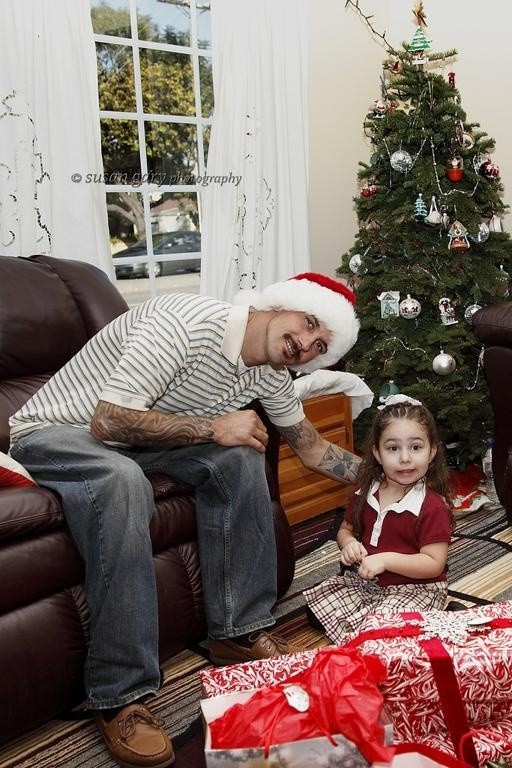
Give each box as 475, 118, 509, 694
112, 229, 201, 278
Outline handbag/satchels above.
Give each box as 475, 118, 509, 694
199, 675, 394, 767
370, 730, 512, 768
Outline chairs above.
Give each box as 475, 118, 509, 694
472, 298, 511, 525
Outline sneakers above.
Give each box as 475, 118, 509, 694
95, 702, 175, 767
209, 632, 305, 661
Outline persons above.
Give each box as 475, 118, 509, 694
4, 270, 359, 767
300, 392, 454, 649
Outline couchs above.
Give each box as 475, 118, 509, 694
0, 254, 296, 747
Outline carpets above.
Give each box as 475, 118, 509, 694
1, 501, 512, 768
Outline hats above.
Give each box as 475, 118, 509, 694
233, 271, 359, 374
